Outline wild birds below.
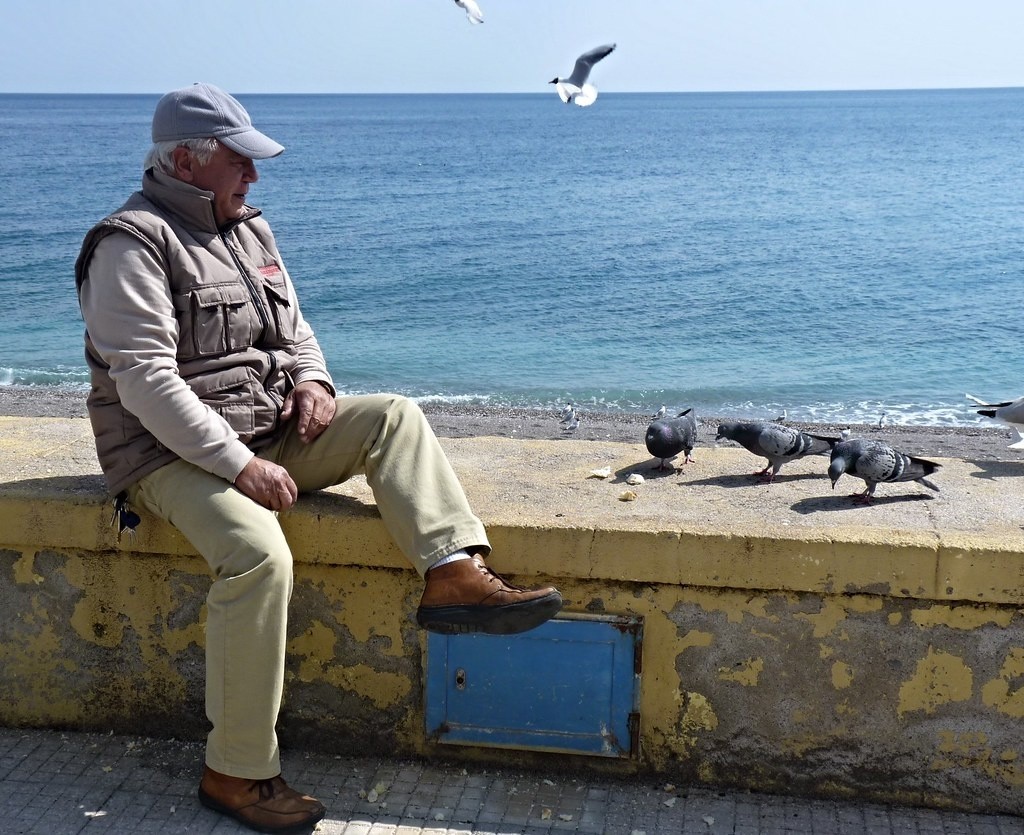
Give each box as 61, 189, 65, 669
771, 409, 787, 423
559, 402, 581, 434
841, 426, 852, 438
547, 43, 615, 108
879, 413, 887, 429
454, 0, 486, 26
963, 393, 1024, 451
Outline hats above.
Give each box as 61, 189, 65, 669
151, 82, 285, 160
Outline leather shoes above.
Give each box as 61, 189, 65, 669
198, 762, 328, 833
417, 552, 563, 635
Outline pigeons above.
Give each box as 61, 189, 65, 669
645, 404, 698, 472
714, 419, 846, 487
827, 439, 943, 507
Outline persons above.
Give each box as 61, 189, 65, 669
68, 81, 565, 835
